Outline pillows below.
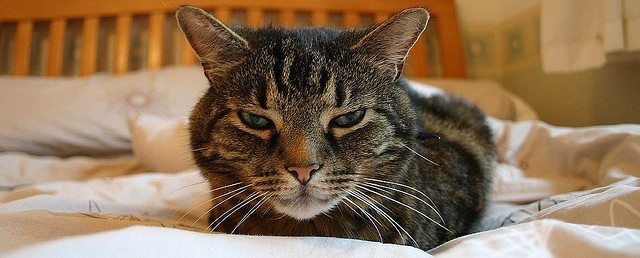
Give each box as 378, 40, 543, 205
0, 65, 213, 158
0, 0, 639, 258
406, 77, 539, 122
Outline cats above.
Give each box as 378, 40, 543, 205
165, 2, 497, 251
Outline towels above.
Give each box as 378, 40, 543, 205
538, 0, 639, 74
